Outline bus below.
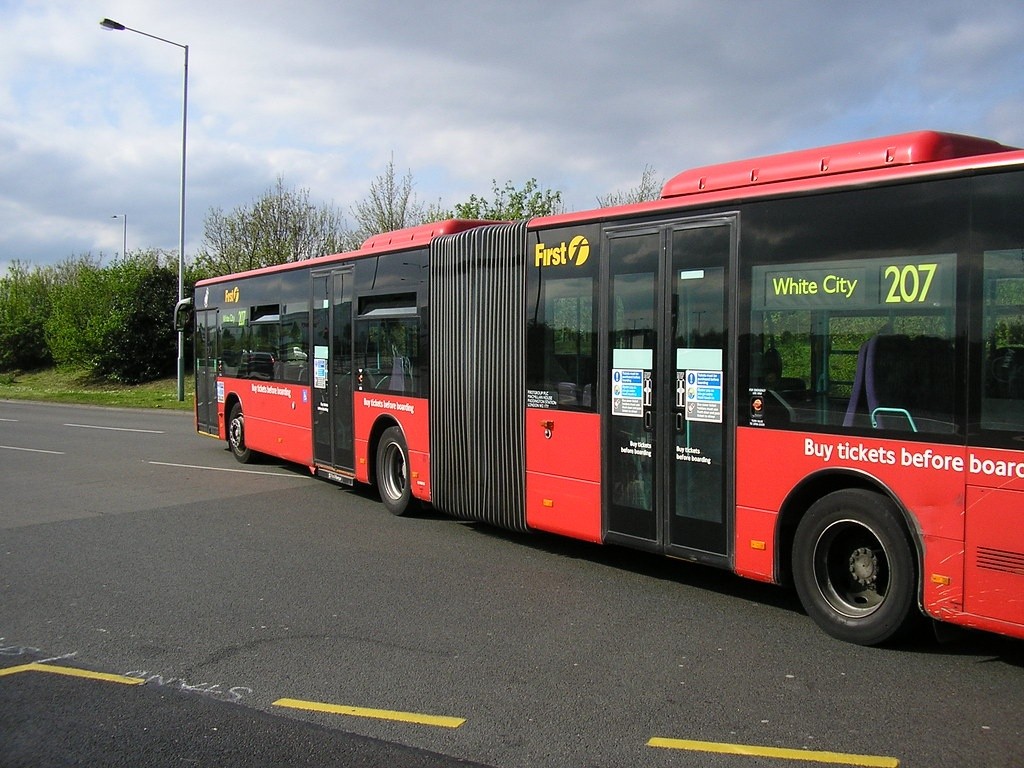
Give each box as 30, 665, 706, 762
246, 307, 419, 395
175, 130, 1024, 646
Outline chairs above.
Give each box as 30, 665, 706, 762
275, 344, 405, 391
765, 334, 954, 436
985, 347, 1024, 400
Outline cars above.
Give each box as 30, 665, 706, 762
247, 352, 274, 379
283, 347, 308, 362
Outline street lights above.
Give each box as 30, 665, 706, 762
111, 214, 127, 261
100, 18, 188, 402
692, 310, 705, 329
627, 318, 643, 330
